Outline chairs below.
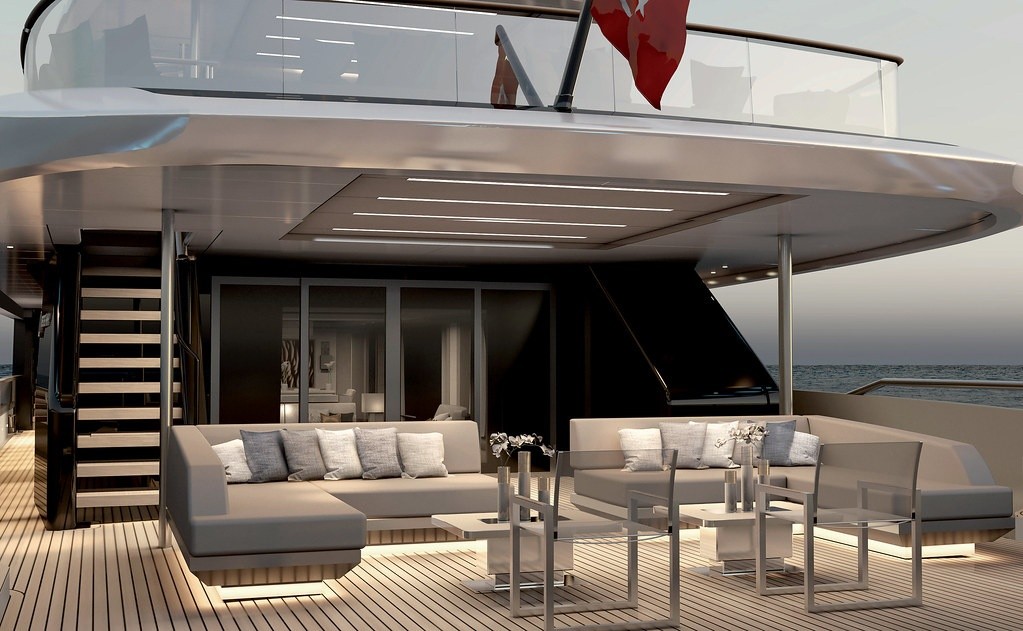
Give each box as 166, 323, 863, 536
338, 389, 356, 403
509, 449, 679, 631
755, 441, 923, 613
427, 403, 467, 421
39, 15, 222, 87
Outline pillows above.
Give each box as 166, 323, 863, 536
618, 420, 821, 472
211, 413, 452, 481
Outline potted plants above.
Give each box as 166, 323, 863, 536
324, 361, 336, 390
281, 360, 292, 392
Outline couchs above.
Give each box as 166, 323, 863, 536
570, 414, 1016, 559
169, 421, 515, 599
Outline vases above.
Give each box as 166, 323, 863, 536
724, 445, 770, 513
498, 451, 549, 520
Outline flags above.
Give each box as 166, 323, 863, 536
491, 25, 521, 109
584, 0, 690, 110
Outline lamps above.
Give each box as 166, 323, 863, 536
361, 393, 384, 421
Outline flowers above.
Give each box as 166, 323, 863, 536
489, 432, 558, 467
714, 423, 770, 459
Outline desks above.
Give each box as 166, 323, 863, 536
431, 500, 804, 594
